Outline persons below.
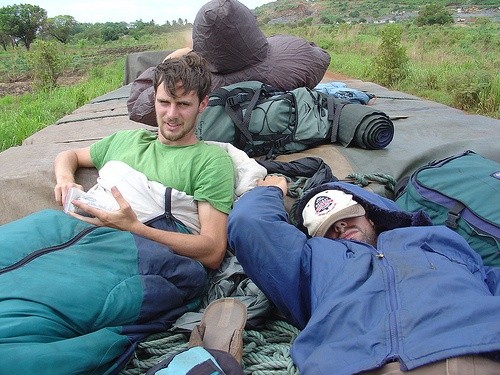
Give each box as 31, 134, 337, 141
54, 54, 234, 270
226, 174, 500, 375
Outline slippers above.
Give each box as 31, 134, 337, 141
188, 298, 247, 371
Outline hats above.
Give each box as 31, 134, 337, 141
302, 190, 366, 240
